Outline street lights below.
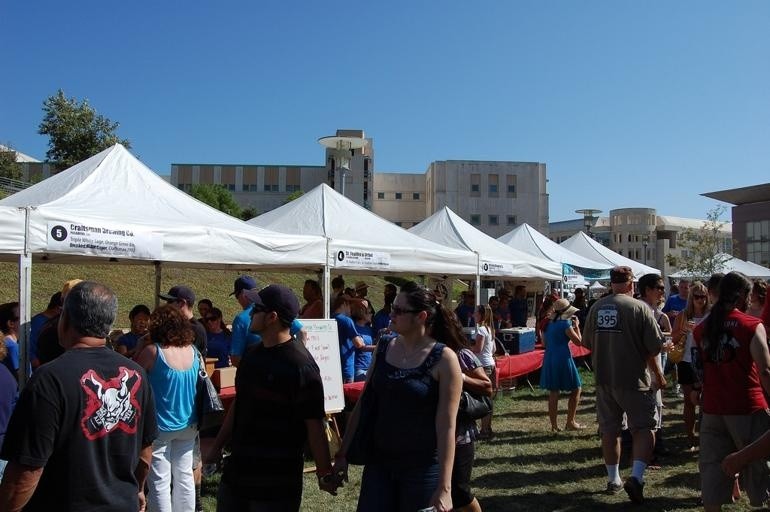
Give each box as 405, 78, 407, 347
315, 130, 369, 294
572, 208, 603, 236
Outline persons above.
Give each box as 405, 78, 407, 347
2, 266, 770, 511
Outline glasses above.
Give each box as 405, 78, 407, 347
166, 299, 179, 304
204, 315, 220, 322
651, 285, 665, 291
252, 306, 271, 314
693, 294, 707, 300
390, 303, 420, 315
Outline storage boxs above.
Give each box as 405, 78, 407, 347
500, 327, 535, 354
214, 367, 236, 388
463, 326, 475, 340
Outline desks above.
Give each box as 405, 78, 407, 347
343, 342, 591, 404
219, 385, 237, 398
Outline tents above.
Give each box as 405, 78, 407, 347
0, 143, 770, 396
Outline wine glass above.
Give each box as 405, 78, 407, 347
665, 337, 672, 354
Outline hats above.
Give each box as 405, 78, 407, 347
242, 282, 301, 319
354, 279, 370, 292
546, 298, 581, 321
156, 284, 197, 306
609, 266, 638, 284
61, 278, 85, 299
227, 275, 258, 297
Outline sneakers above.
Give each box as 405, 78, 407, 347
605, 480, 625, 496
622, 475, 647, 506
552, 426, 565, 432
565, 423, 587, 431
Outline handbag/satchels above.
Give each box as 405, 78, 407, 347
195, 345, 228, 431
455, 343, 494, 422
666, 309, 688, 365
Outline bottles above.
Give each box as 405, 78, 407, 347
200, 450, 222, 477
